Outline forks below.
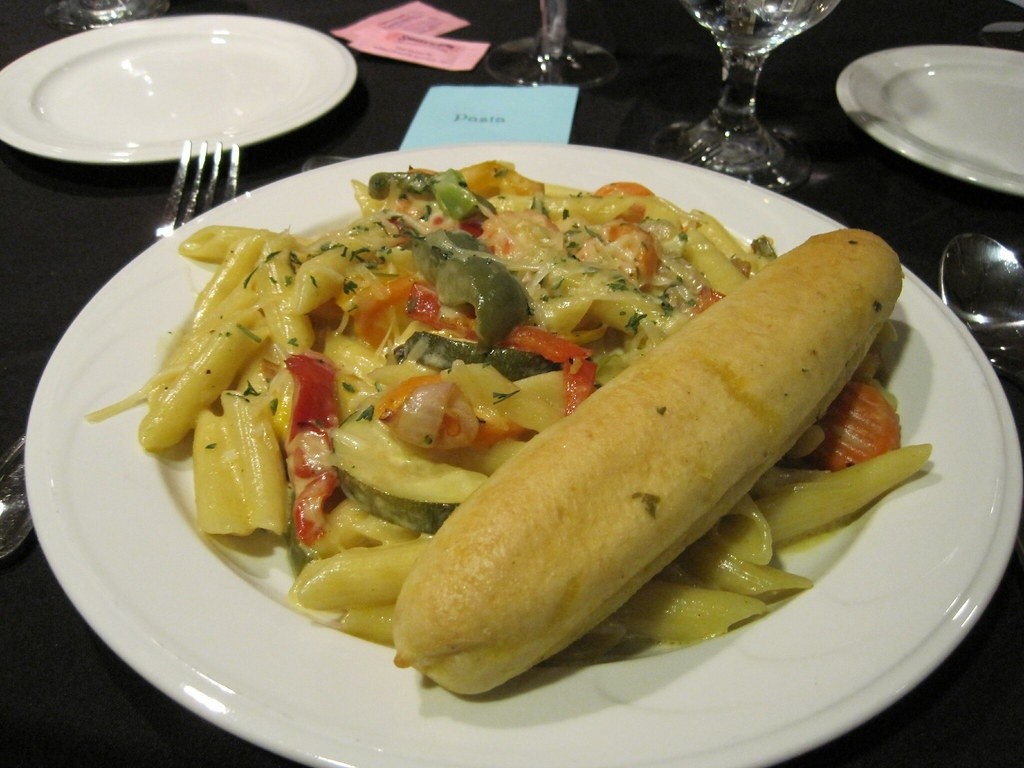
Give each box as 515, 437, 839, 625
0, 141, 240, 566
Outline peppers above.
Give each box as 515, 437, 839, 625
563, 360, 596, 417
410, 280, 590, 360
287, 355, 337, 546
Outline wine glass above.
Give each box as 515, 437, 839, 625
651, 0, 842, 193
478, 0, 620, 91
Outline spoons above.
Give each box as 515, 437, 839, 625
939, 231, 1024, 391
978, 20, 1024, 52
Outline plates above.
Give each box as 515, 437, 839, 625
0, 14, 358, 165
22, 143, 1024, 768
835, 45, 1024, 196
